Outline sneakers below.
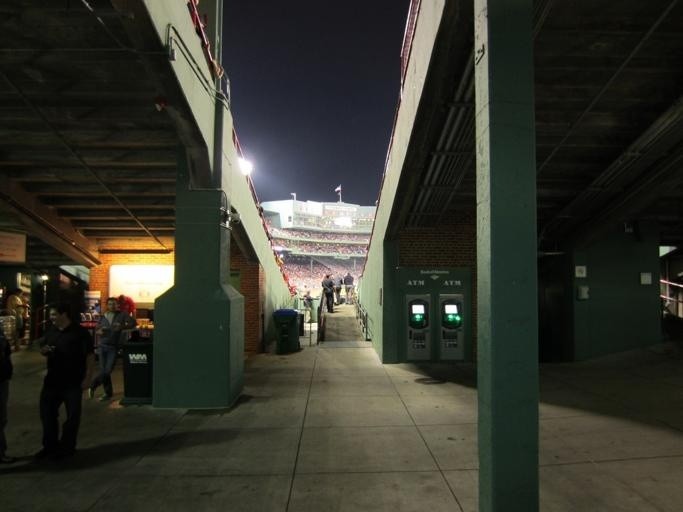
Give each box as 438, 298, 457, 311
86, 387, 112, 402
33, 439, 78, 461
0, 454, 18, 465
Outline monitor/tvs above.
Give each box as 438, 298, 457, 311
444, 305, 458, 314
411, 304, 425, 314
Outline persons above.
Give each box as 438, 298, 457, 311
39, 303, 94, 462
0, 323, 15, 465
333, 275, 344, 305
303, 290, 313, 323
344, 272, 354, 305
88, 295, 137, 401
7, 288, 30, 352
321, 274, 335, 313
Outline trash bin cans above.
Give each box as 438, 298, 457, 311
272, 309, 305, 353
119, 328, 152, 405
